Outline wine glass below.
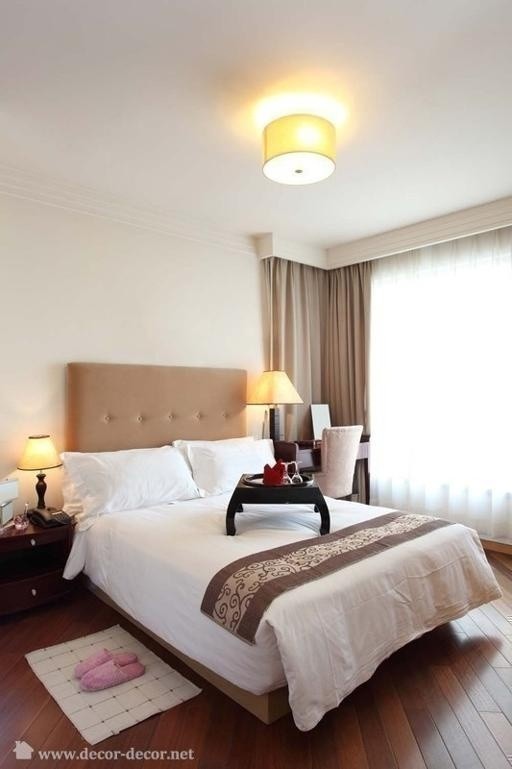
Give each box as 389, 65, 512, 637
290, 461, 303, 484
280, 462, 293, 485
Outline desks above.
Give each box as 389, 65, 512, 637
274, 435, 371, 506
226, 472, 330, 535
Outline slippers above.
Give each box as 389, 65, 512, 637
73, 648, 145, 693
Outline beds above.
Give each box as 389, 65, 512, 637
59, 359, 502, 733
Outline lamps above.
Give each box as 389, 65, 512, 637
261, 115, 338, 184
17, 433, 64, 509
244, 370, 303, 439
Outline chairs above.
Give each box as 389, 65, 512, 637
311, 425, 365, 502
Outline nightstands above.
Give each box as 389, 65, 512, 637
0, 516, 74, 618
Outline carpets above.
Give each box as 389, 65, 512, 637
25, 625, 202, 746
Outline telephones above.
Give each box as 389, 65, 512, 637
27, 507, 71, 528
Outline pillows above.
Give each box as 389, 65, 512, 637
59, 436, 277, 521
